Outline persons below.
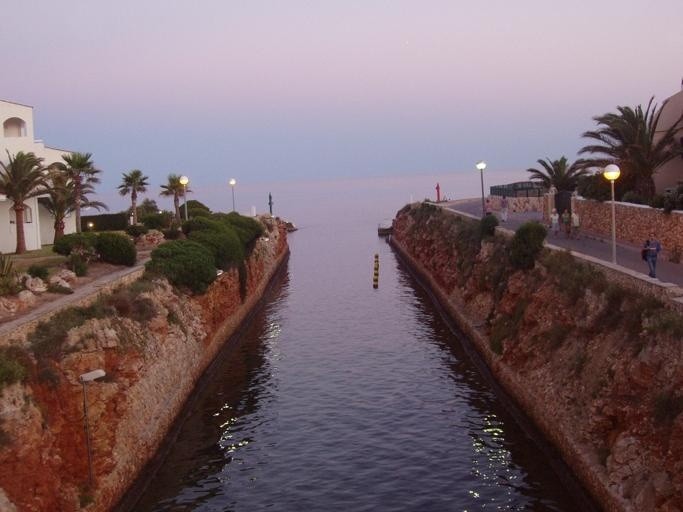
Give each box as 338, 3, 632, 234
484, 198, 494, 216
641, 231, 661, 278
560, 208, 571, 237
569, 208, 580, 240
548, 207, 561, 240
498, 193, 510, 223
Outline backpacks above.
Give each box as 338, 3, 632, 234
640, 240, 650, 261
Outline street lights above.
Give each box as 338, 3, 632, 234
604, 164, 620, 264
179, 176, 189, 221
230, 178, 236, 211
477, 161, 486, 215
